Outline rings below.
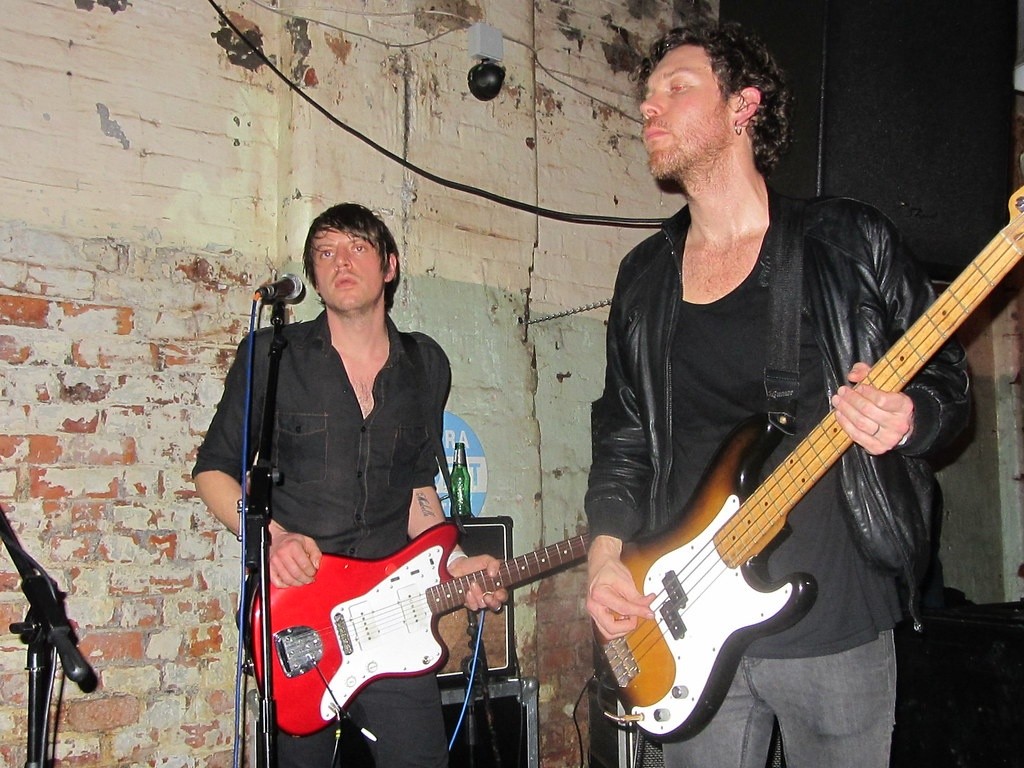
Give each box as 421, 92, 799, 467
492, 605, 501, 611
872, 424, 880, 435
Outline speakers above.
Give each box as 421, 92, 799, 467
434, 516, 513, 684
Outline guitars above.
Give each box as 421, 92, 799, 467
590, 186, 1024, 744
234, 522, 602, 739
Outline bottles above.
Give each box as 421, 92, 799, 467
450, 443, 471, 518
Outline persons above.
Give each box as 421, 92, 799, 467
192, 202, 509, 768
584, 21, 977, 768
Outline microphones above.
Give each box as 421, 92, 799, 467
255, 272, 303, 299
